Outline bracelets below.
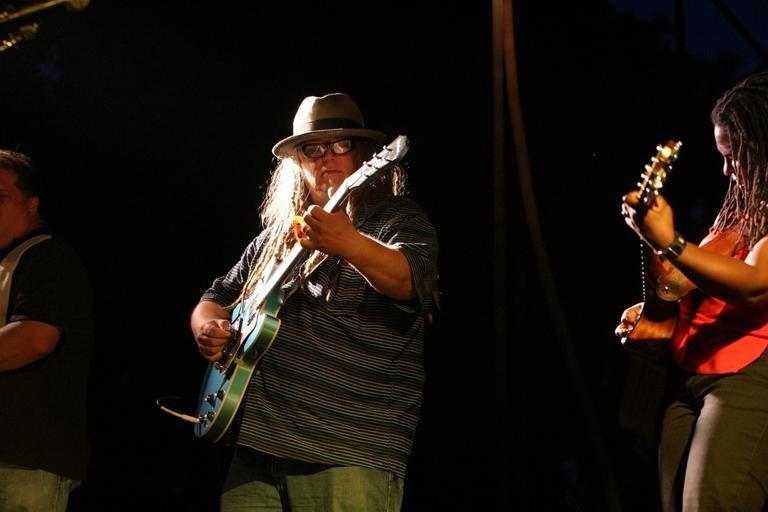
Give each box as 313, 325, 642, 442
653, 232, 686, 262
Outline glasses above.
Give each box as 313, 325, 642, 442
301, 139, 353, 157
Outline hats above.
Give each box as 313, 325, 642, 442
271, 93, 385, 159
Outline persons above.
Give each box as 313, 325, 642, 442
615, 71, 768, 512
0, 149, 77, 511
190, 92, 439, 512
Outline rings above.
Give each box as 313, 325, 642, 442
624, 205, 627, 216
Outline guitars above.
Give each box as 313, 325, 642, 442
194, 134, 411, 440
627, 140, 684, 341
